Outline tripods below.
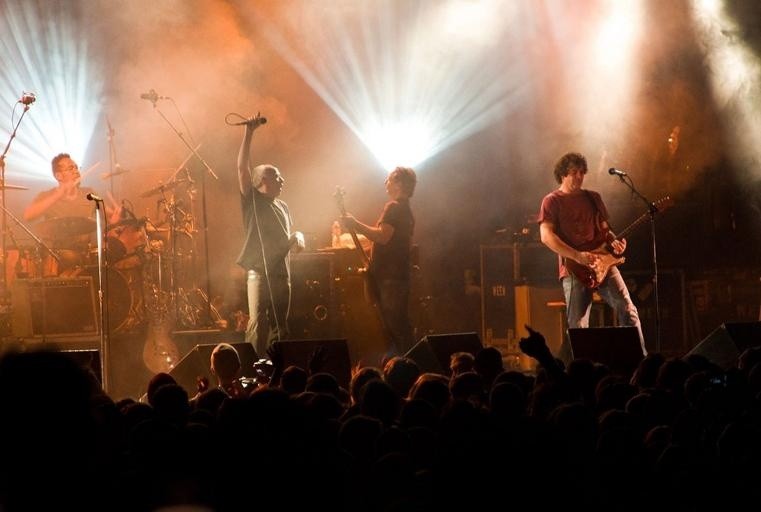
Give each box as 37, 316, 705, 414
114, 188, 223, 332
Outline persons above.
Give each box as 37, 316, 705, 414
337, 165, 418, 366
236, 109, 307, 362
536, 152, 650, 360
0, 323, 761, 511
23, 152, 126, 228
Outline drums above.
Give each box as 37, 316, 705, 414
35, 246, 85, 281
74, 264, 133, 332
147, 228, 195, 295
105, 216, 156, 270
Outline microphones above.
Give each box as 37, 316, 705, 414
609, 168, 627, 176
20, 93, 36, 104
84, 191, 102, 206
100, 167, 129, 180
141, 93, 170, 100
241, 117, 267, 125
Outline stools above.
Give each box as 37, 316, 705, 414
546, 297, 608, 345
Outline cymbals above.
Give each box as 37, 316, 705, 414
37, 214, 99, 238
143, 177, 183, 199
1, 185, 28, 191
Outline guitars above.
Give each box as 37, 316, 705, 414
332, 187, 376, 301
563, 195, 674, 290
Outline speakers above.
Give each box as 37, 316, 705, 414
560, 326, 643, 372
51, 332, 484, 397
679, 320, 761, 370
